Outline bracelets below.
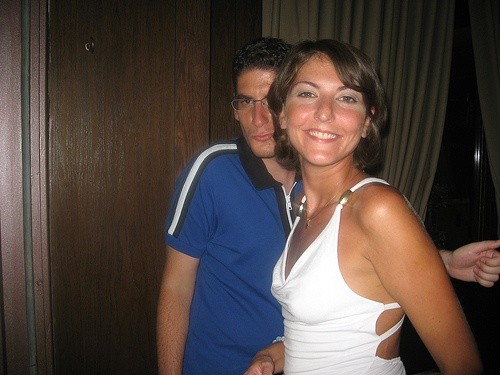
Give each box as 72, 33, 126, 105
272, 336, 286, 343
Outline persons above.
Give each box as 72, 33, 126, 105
245, 38, 482, 375
156, 37, 500, 375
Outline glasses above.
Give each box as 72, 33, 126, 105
230, 96, 269, 113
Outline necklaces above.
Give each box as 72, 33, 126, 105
301, 168, 371, 230
